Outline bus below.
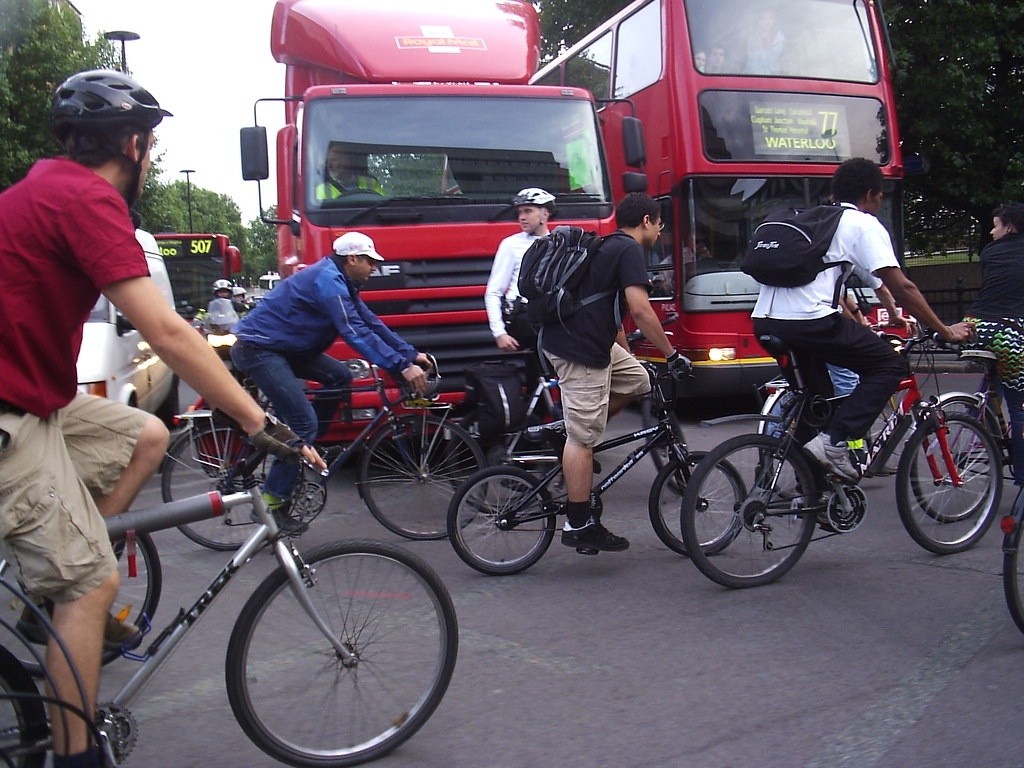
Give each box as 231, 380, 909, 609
528, 0, 911, 401
152, 232, 242, 323
258, 274, 281, 294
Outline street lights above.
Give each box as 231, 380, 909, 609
179, 168, 197, 233
103, 31, 141, 74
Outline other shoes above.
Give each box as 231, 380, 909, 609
250, 505, 308, 532
18, 599, 142, 652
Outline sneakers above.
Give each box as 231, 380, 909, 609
803, 431, 859, 484
793, 493, 828, 523
592, 459, 601, 475
561, 516, 629, 551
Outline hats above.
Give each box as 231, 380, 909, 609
333, 233, 385, 261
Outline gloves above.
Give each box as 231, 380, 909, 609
666, 350, 693, 382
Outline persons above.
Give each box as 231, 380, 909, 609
0, 69, 326, 767
969, 203, 1024, 487
750, 156, 978, 485
659, 229, 712, 293
693, 43, 725, 76
541, 180, 709, 552
730, 4, 791, 77
192, 278, 247, 334
484, 187, 603, 492
230, 232, 434, 534
317, 142, 384, 204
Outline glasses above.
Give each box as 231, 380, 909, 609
652, 217, 664, 230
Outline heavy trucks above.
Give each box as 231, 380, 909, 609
240, 0, 648, 444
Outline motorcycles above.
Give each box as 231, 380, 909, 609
194, 298, 244, 372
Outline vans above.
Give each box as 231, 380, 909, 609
77, 228, 180, 430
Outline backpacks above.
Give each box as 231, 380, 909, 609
740, 202, 858, 288
517, 226, 639, 326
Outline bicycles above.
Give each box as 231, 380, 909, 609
157, 352, 489, 551
446, 312, 748, 576
1000, 485, 1024, 633
0, 419, 458, 768
679, 317, 1018, 588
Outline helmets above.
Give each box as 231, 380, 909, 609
51, 70, 174, 143
212, 279, 233, 297
513, 188, 556, 206
231, 287, 247, 295
